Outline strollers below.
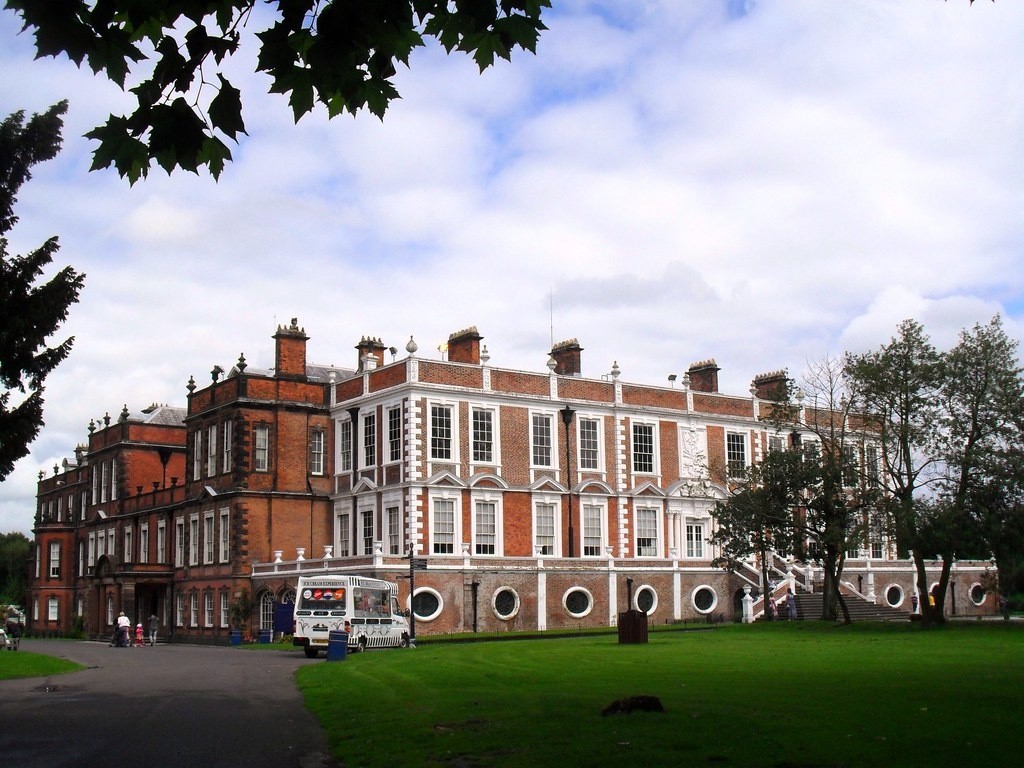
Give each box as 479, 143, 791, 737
109, 623, 132, 648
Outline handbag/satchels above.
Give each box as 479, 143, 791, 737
786, 603, 790, 609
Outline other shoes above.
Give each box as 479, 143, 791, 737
151, 642, 154, 646
788, 619, 793, 621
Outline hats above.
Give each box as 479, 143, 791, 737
120, 612, 126, 616
137, 624, 142, 627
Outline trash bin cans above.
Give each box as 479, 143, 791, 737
258, 629, 271, 644
325, 629, 349, 662
229, 628, 242, 646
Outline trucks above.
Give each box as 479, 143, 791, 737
293, 575, 412, 658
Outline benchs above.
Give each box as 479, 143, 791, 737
0, 633, 18, 651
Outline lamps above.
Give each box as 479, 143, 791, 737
667, 374, 676, 389
437, 344, 447, 361
96, 419, 101, 432
216, 365, 225, 381
389, 347, 397, 362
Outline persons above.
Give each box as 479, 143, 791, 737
768, 577, 777, 591
786, 588, 797, 621
911, 592, 918, 612
929, 592, 934, 604
117, 612, 160, 648
768, 593, 778, 622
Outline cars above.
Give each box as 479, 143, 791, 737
0, 629, 11, 648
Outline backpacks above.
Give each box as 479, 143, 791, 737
151, 618, 158, 631
